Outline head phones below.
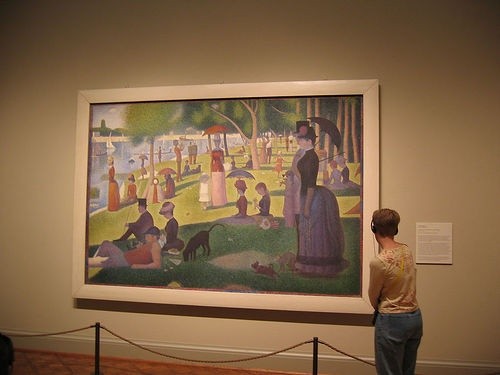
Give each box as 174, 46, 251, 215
370, 218, 398, 235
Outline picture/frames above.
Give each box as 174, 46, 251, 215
73, 78, 380, 314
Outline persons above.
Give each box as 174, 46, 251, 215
368, 208, 423, 375
